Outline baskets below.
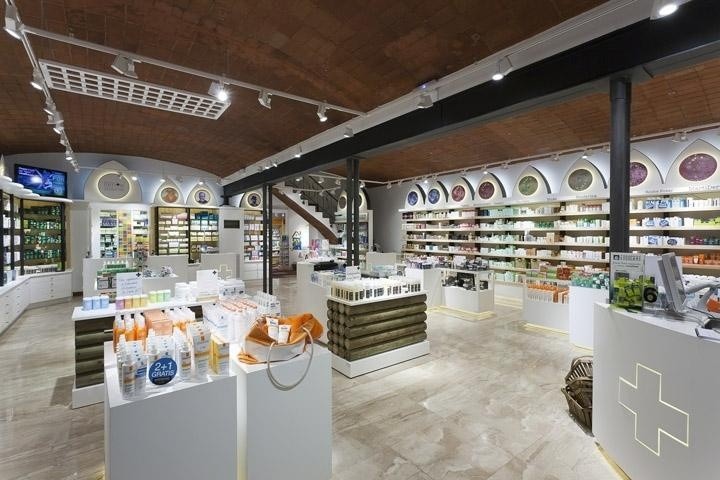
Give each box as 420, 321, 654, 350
560, 355, 592, 430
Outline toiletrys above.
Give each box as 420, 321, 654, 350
278, 324, 291, 346
113, 290, 282, 402
330, 276, 421, 302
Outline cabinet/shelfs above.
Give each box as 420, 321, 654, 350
0, 174, 720, 480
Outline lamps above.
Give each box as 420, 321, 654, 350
0, 0, 720, 186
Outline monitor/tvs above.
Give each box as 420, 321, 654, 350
657, 251, 688, 314
14, 164, 67, 198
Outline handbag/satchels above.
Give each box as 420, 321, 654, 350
244, 317, 314, 363
